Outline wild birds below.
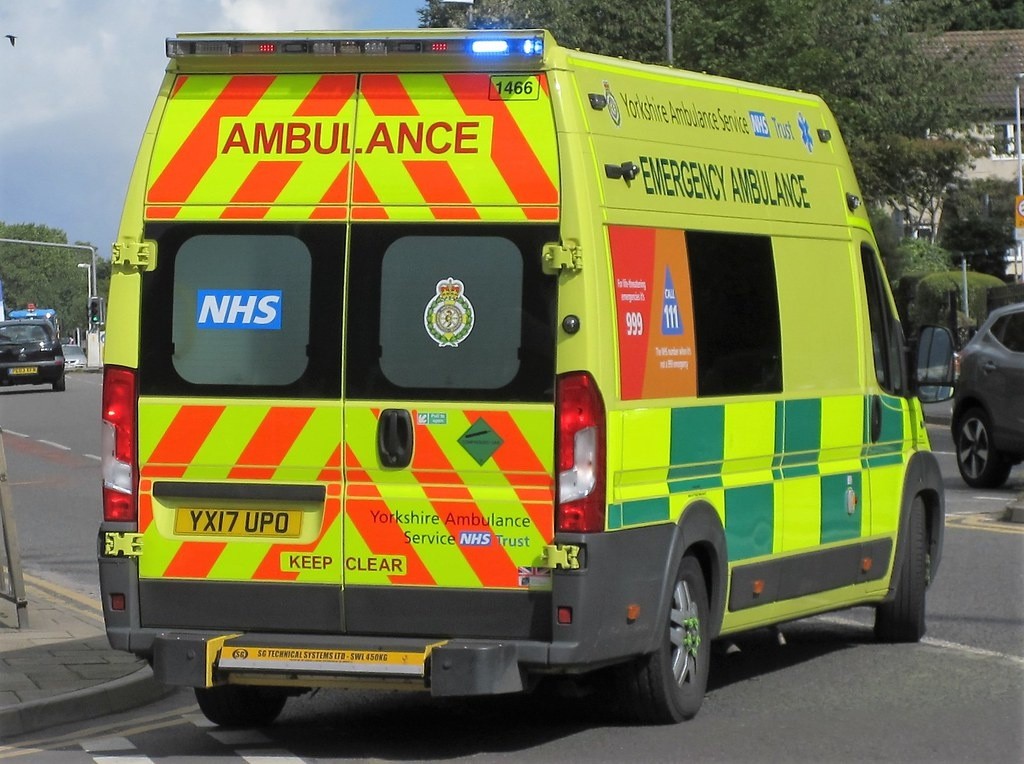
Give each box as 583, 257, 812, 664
5, 35, 17, 47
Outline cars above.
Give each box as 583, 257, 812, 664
0, 319, 66, 391
59, 337, 74, 346
62, 344, 86, 371
952, 302, 1024, 490
8, 303, 56, 336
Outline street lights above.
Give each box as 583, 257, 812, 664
77, 263, 92, 331
1013, 72, 1024, 258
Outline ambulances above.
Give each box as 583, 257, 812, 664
97, 15, 952, 725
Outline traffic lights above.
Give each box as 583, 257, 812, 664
91, 300, 99, 322
